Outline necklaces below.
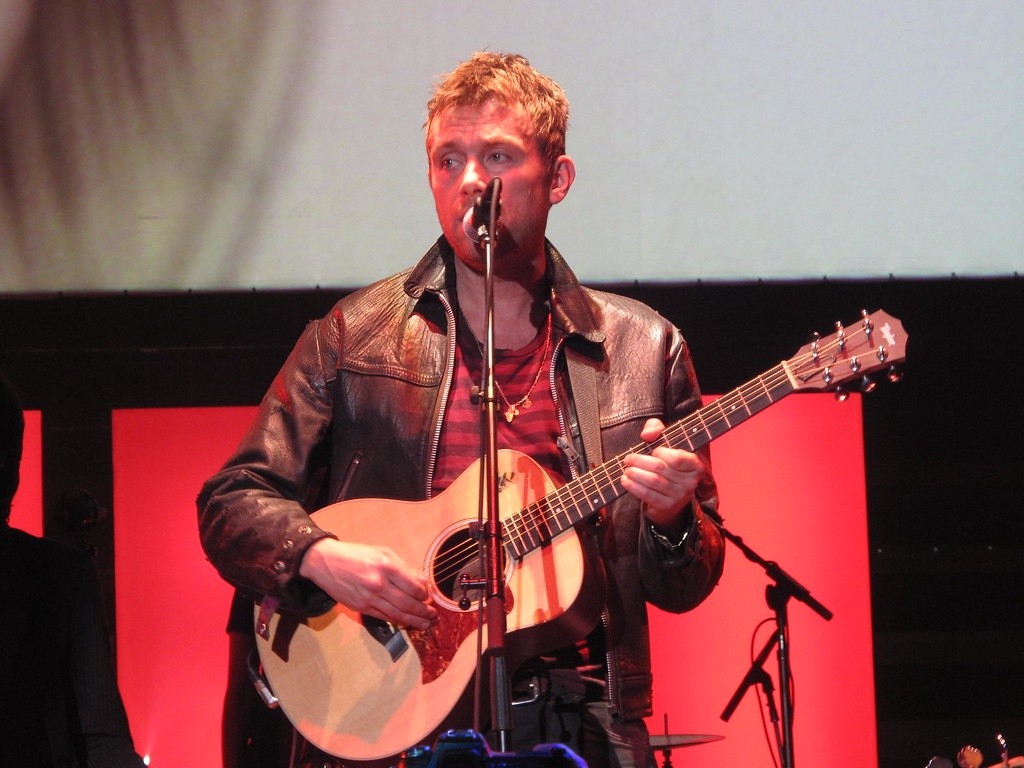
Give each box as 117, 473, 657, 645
461, 300, 552, 422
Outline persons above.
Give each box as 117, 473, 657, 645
197, 50, 725, 768
0, 377, 146, 768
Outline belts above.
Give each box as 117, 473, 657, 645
508, 667, 607, 706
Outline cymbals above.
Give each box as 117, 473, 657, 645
649, 733, 727, 748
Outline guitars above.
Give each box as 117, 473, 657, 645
245, 306, 915, 765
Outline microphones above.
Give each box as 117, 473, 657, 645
719, 629, 780, 721
463, 177, 501, 245
